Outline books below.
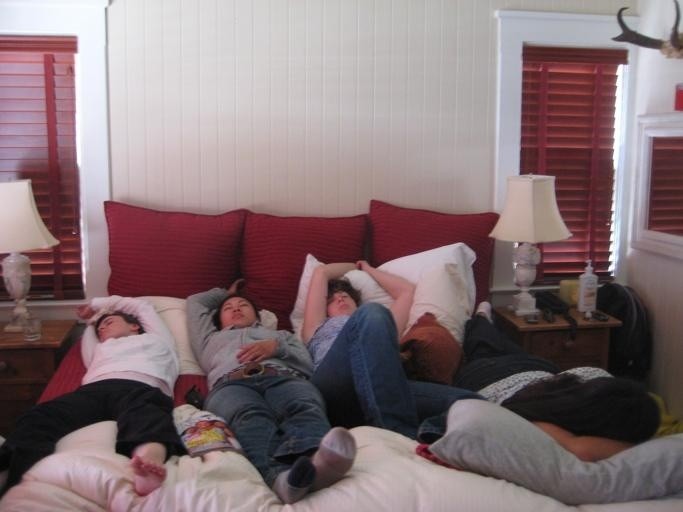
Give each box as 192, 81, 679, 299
175, 414, 241, 457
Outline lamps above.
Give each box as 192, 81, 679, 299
0, 180, 61, 330
487, 173, 573, 316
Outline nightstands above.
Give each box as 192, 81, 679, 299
0, 319, 81, 436
498, 304, 621, 378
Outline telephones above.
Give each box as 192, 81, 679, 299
537, 291, 571, 312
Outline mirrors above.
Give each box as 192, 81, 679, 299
630, 111, 683, 259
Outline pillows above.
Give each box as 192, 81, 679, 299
101, 202, 244, 299
367, 201, 498, 301
239, 205, 369, 299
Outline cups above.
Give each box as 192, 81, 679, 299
23, 318, 42, 342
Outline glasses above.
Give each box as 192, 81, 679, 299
185, 385, 204, 409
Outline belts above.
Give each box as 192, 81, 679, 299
217, 361, 302, 385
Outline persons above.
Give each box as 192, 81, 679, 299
0, 293, 185, 498
184, 419, 233, 440
301, 260, 491, 443
453, 300, 659, 460
184, 276, 357, 507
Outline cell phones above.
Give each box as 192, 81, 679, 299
593, 312, 609, 321
528, 315, 538, 322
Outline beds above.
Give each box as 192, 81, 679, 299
0, 301, 517, 510
